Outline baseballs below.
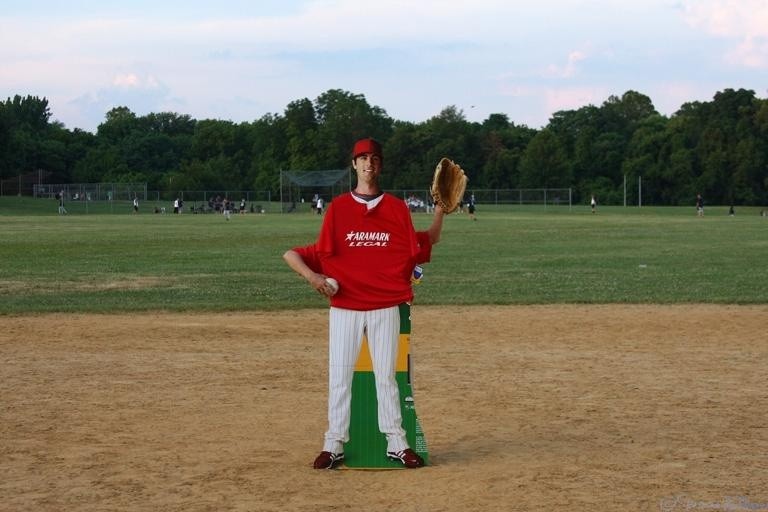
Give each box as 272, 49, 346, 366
325, 278, 339, 297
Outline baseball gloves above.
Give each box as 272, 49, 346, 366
431, 158, 468, 214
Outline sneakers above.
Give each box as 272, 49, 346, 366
386, 448, 424, 468
314, 451, 345, 470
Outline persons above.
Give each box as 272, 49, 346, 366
313, 138, 424, 470
76, 192, 91, 200
553, 191, 560, 205
591, 196, 595, 214
133, 197, 138, 215
283, 204, 444, 296
311, 198, 325, 215
408, 194, 476, 220
729, 205, 735, 216
173, 195, 246, 221
696, 195, 704, 216
153, 206, 160, 213
108, 192, 112, 200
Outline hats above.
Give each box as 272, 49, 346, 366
353, 139, 382, 158
